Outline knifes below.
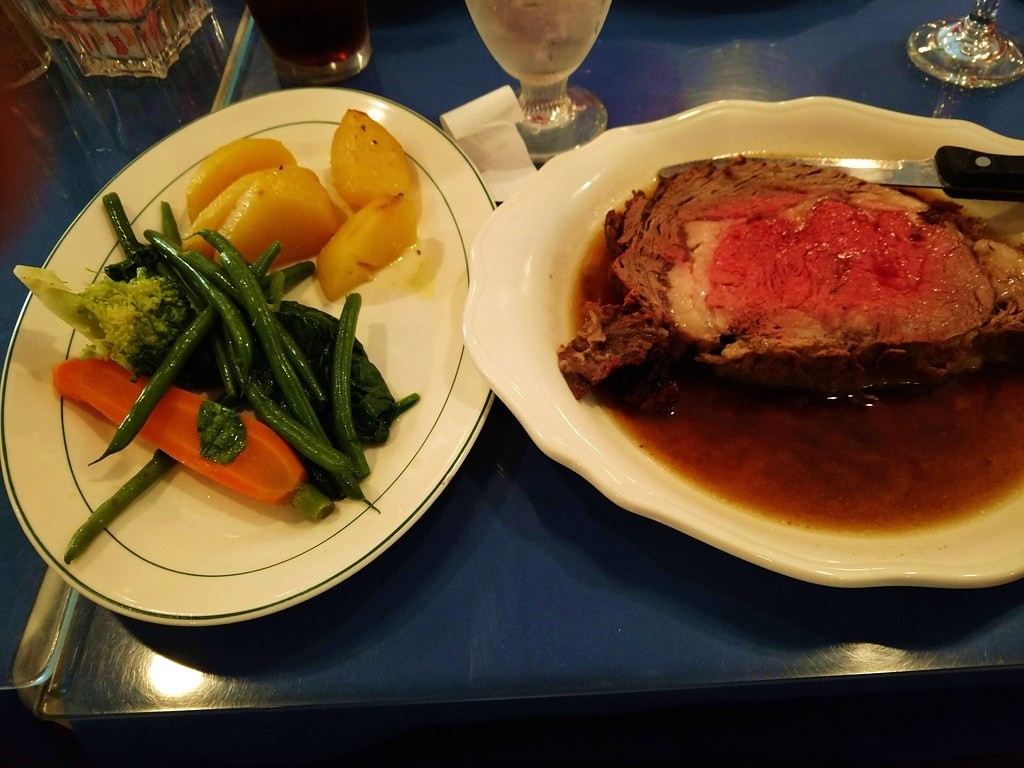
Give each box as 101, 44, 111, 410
658, 145, 1024, 202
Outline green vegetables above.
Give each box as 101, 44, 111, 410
197, 303, 420, 464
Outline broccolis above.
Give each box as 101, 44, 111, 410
13, 265, 194, 382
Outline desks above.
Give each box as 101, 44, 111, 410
0, 0, 255, 691
41, 0, 1024, 741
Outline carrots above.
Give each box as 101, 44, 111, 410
53, 359, 302, 502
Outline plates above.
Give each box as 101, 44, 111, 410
464, 95, 1024, 586
0, 88, 498, 626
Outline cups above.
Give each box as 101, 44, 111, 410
245, 0, 372, 86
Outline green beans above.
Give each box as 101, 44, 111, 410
64, 189, 370, 569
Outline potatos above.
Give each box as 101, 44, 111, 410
180, 109, 423, 303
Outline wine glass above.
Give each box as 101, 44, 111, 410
465, 0, 612, 164
907, 0, 1024, 90
